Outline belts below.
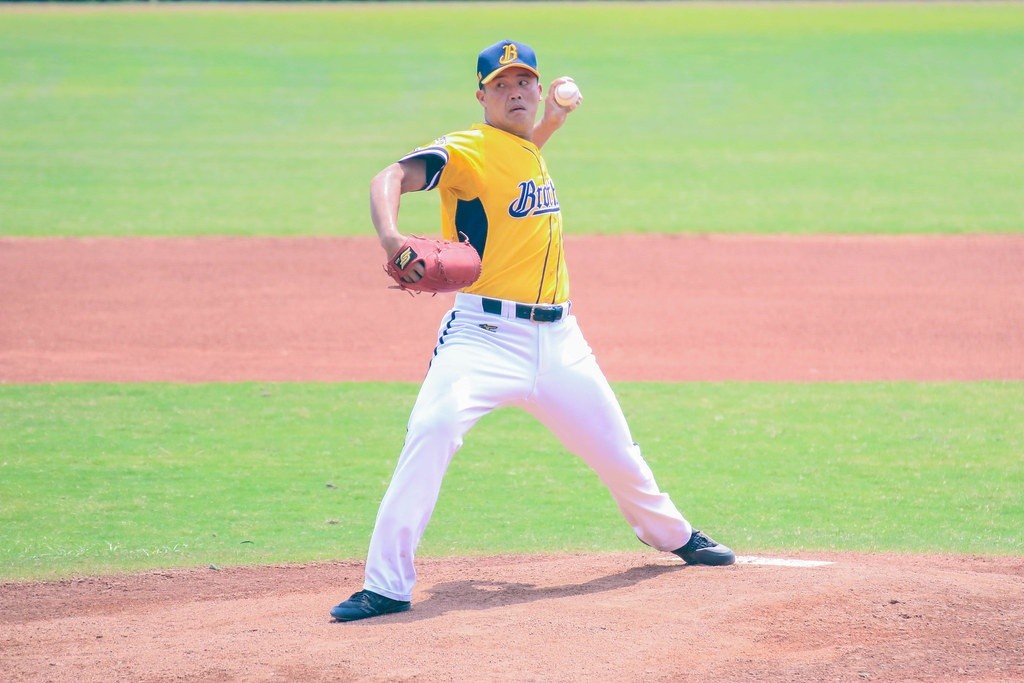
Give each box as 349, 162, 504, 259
482, 297, 570, 322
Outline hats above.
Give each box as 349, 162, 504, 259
477, 39, 540, 90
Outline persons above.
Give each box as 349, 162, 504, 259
326, 39, 735, 621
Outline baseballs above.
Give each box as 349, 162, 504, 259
554, 81, 581, 108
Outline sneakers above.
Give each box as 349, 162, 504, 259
329, 588, 411, 621
637, 527, 735, 566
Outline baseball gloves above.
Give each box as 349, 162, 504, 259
379, 230, 484, 300
